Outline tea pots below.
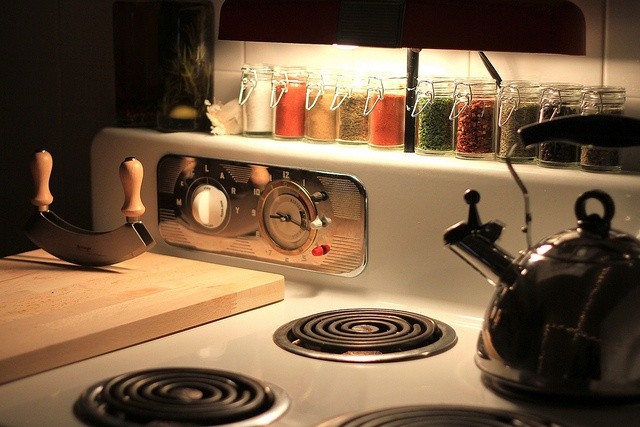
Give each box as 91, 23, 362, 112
439, 185, 640, 410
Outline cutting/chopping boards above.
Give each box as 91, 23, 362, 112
0, 233, 286, 386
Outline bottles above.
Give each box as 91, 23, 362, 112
539, 81, 584, 169
272, 67, 308, 140
415, 77, 458, 156
304, 72, 340, 142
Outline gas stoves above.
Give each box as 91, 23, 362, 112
0, 277, 640, 427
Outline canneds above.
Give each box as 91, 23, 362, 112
413, 79, 453, 155
544, 82, 580, 166
307, 74, 336, 140
275, 66, 305, 139
